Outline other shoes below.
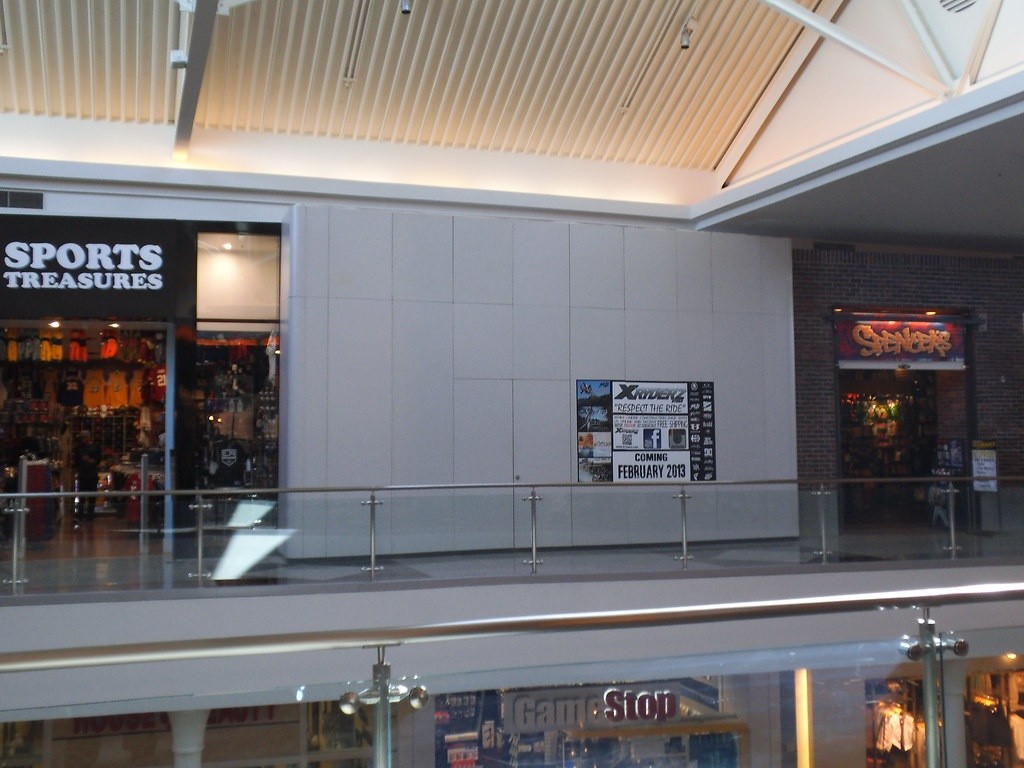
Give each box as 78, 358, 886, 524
86, 517, 94, 524
72, 517, 82, 528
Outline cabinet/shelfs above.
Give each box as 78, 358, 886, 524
0, 327, 278, 528
840, 392, 970, 526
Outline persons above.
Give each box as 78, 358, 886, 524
74, 428, 102, 523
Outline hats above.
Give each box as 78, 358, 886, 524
78, 430, 89, 437
